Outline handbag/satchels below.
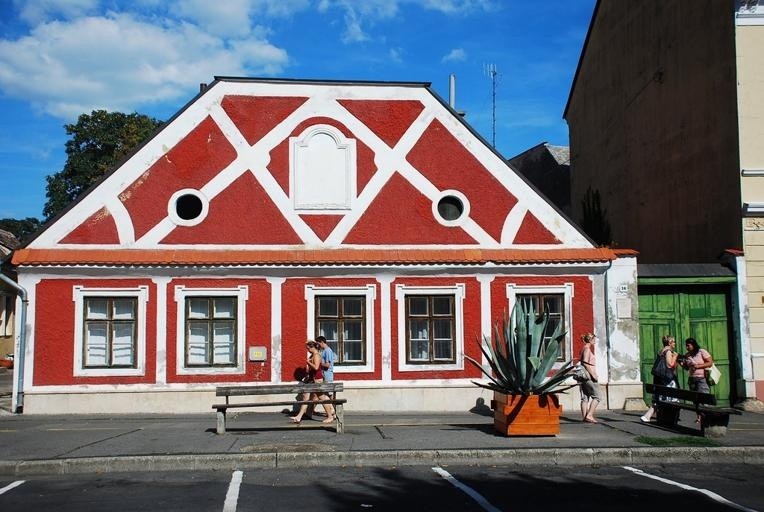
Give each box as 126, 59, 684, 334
573, 365, 591, 381
705, 363, 722, 386
651, 354, 674, 384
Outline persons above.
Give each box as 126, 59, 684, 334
677, 337, 715, 422
288, 340, 334, 423
579, 332, 602, 424
640, 334, 682, 423
301, 334, 339, 420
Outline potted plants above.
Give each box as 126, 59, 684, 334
460, 297, 587, 438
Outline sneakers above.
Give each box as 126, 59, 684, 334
641, 416, 651, 422
696, 420, 701, 424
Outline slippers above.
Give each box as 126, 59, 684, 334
586, 417, 597, 423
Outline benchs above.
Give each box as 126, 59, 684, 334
642, 383, 739, 436
212, 383, 348, 434
